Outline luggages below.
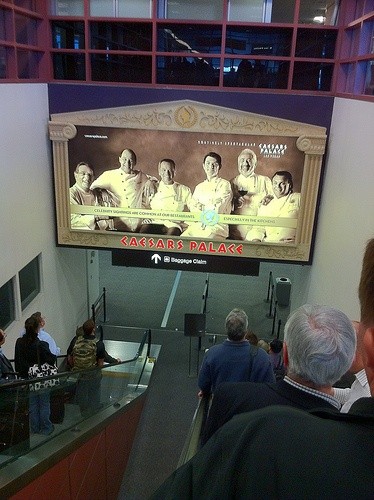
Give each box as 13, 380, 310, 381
49, 383, 65, 425
0, 371, 28, 451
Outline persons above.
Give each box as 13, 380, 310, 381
0, 312, 121, 436
149, 240, 374, 500
198, 306, 372, 446
69, 149, 301, 244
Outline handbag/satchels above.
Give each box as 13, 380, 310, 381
28, 341, 61, 392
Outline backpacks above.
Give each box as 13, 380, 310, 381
72, 337, 100, 368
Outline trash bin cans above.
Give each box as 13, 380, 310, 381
276, 277, 292, 306
